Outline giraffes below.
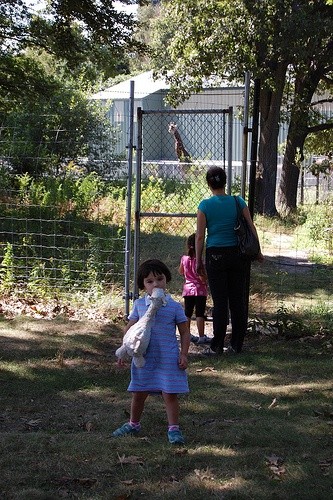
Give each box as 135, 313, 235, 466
168, 121, 192, 163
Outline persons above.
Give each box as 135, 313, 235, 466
196, 165, 264, 355
111, 259, 189, 444
179, 231, 210, 343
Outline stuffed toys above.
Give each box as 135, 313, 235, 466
115, 287, 167, 367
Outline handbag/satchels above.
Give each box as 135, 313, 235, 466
233, 195, 261, 261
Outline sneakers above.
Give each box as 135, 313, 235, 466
189, 334, 199, 342
168, 430, 184, 444
226, 342, 237, 353
113, 423, 141, 437
197, 335, 212, 344
202, 347, 220, 356
